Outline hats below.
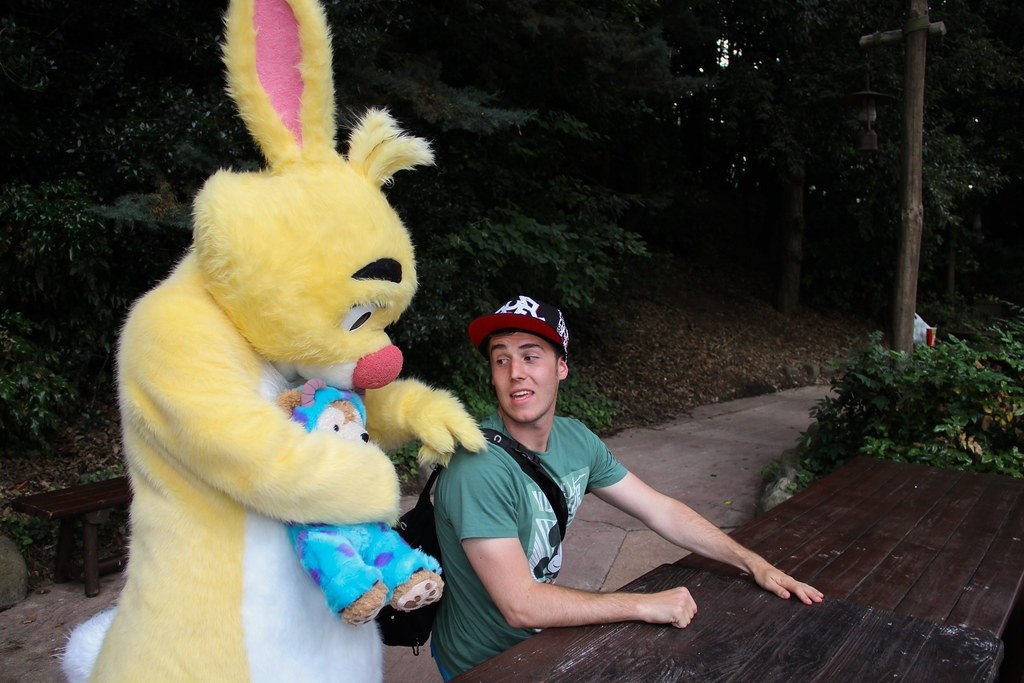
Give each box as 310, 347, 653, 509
468, 294, 570, 364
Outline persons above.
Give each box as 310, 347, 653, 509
431, 297, 825, 683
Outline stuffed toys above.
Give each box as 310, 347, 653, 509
57, 1, 487, 683
275, 379, 445, 626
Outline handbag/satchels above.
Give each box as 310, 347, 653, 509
377, 498, 444, 647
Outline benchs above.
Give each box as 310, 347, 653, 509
11, 476, 136, 598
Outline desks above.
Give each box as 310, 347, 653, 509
444, 455, 1024, 683
